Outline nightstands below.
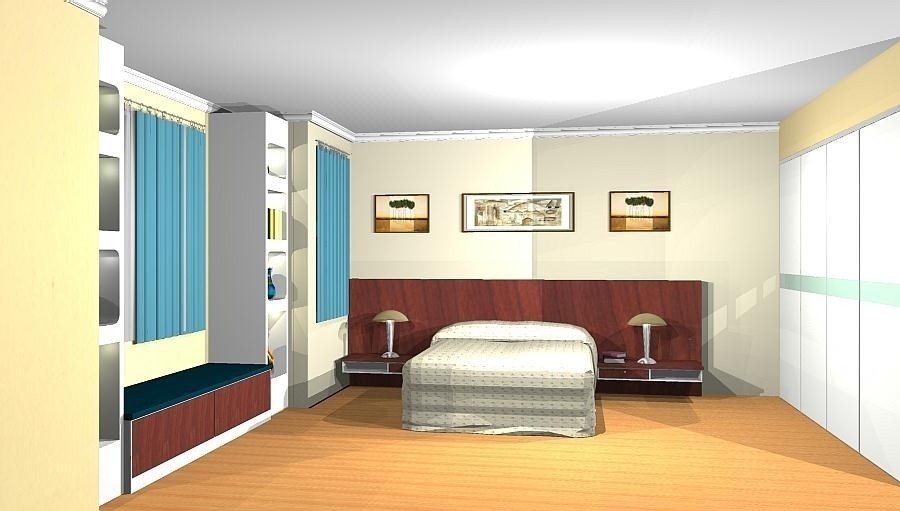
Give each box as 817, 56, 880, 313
339, 353, 409, 387
598, 361, 704, 395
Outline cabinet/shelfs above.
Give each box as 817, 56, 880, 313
127, 361, 273, 500
208, 109, 291, 416
98, 33, 125, 510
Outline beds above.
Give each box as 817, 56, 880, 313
399, 319, 605, 440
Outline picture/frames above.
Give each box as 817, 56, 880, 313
371, 189, 673, 234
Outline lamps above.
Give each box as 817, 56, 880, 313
625, 312, 668, 366
372, 308, 409, 360
